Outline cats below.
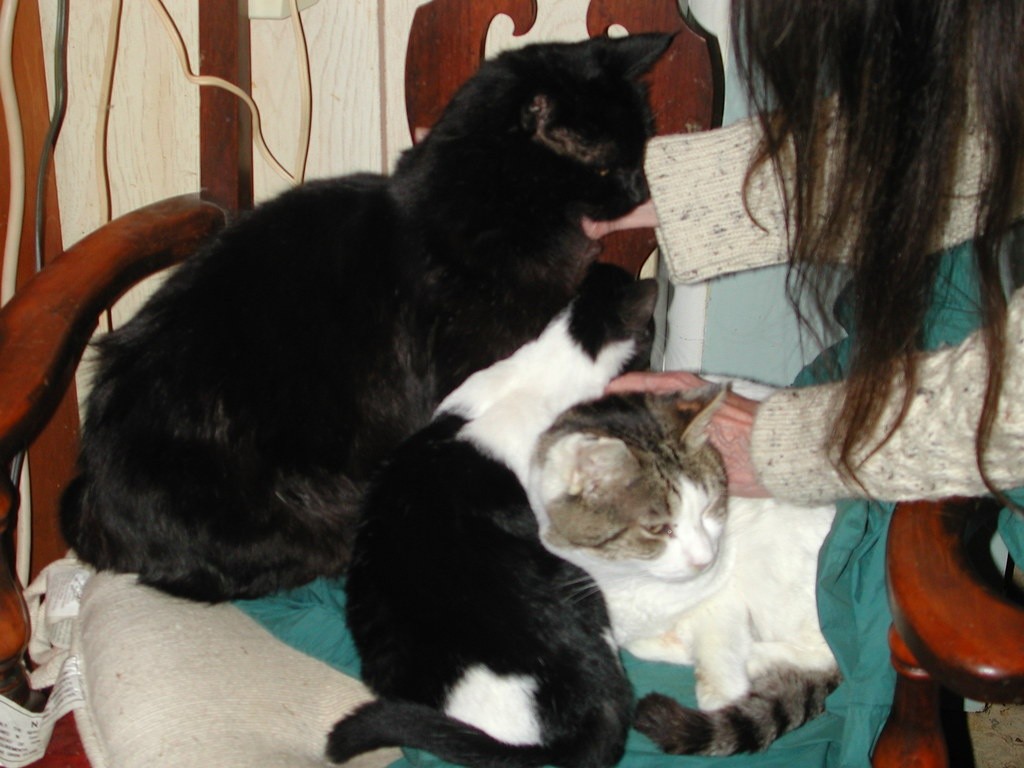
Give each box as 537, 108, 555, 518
51, 26, 843, 766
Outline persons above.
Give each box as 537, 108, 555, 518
581, 0, 1024, 505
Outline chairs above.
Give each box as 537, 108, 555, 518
0, 0, 1024, 768
